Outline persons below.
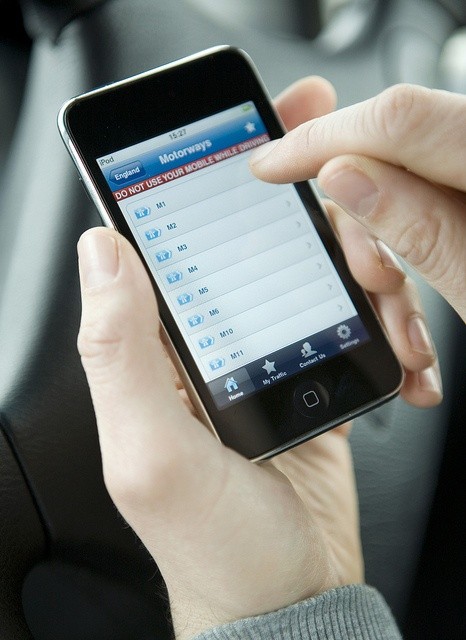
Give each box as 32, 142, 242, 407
74, 73, 466, 639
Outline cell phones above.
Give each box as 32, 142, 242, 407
55, 44, 406, 464
